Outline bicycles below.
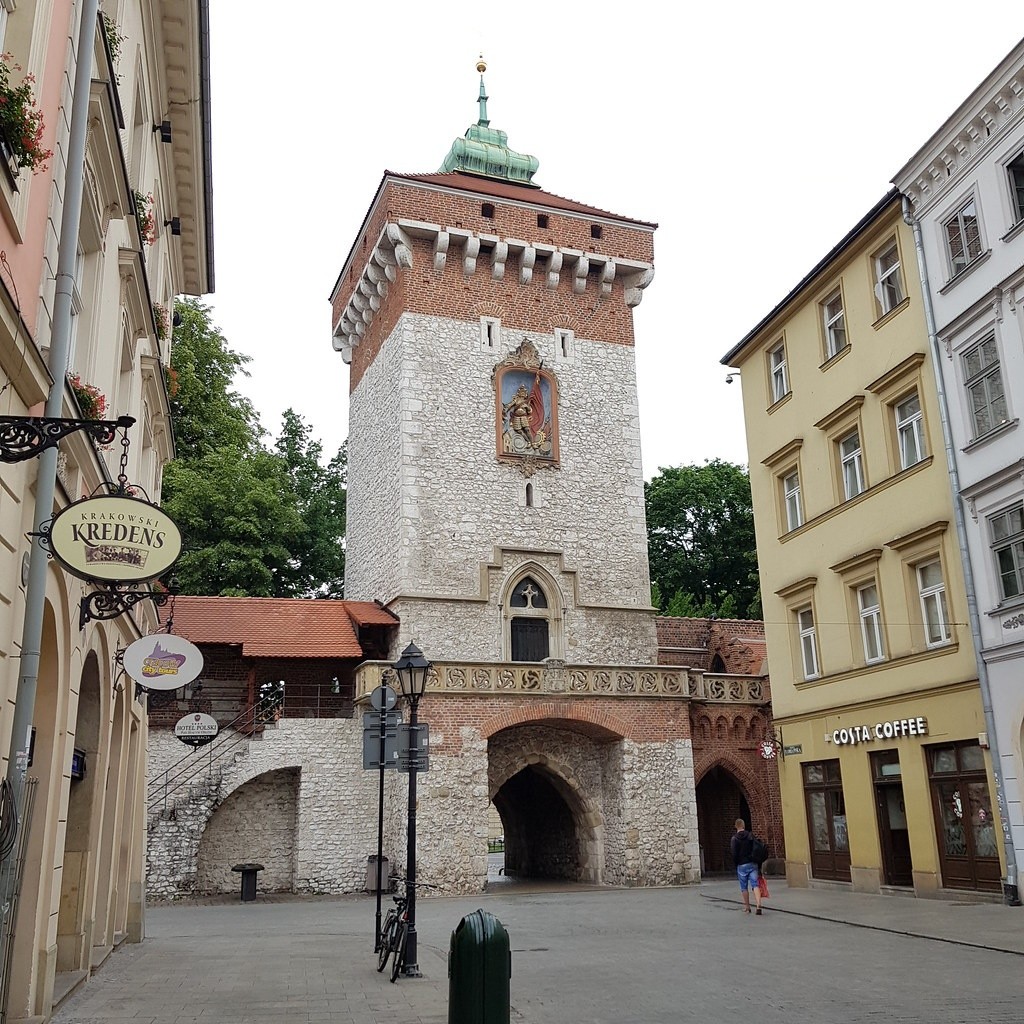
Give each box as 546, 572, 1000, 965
375, 875, 439, 984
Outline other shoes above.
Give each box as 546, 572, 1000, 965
755, 904, 763, 915
742, 906, 751, 913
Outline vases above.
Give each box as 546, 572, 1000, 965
0, 137, 13, 163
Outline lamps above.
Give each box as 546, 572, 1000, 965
153, 120, 173, 144
164, 216, 181, 236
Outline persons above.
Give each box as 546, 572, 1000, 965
730, 820, 764, 915
500, 385, 540, 449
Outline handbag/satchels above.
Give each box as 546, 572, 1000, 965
752, 874, 769, 898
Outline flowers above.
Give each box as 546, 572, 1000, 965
153, 302, 172, 342
66, 370, 115, 454
0, 51, 54, 176
153, 581, 169, 593
163, 363, 181, 401
136, 189, 158, 246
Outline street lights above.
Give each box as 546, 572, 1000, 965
386, 638, 435, 979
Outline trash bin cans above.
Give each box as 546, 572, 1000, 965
447, 908, 512, 1024
367, 855, 389, 896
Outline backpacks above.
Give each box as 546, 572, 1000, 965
746, 831, 763, 864
